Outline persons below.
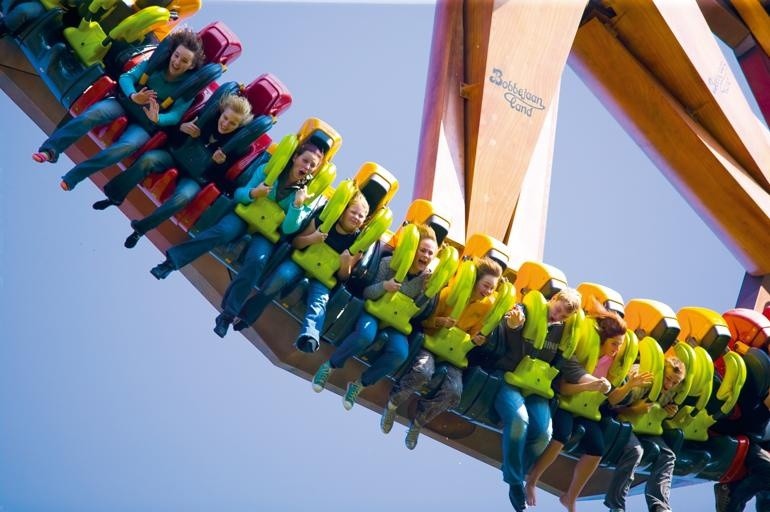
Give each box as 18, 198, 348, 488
603, 355, 687, 511
232, 194, 371, 354
92, 94, 256, 248
0, 1, 45, 38
150, 144, 323, 339
380, 256, 503, 450
32, 28, 207, 191
524, 310, 654, 512
713, 336, 770, 512
311, 224, 438, 412
492, 286, 612, 511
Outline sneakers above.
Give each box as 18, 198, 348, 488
380, 402, 397, 434
343, 377, 363, 411
312, 359, 336, 393
213, 311, 234, 338
714, 483, 731, 512
509, 484, 527, 512
405, 418, 420, 450
149, 260, 175, 280
